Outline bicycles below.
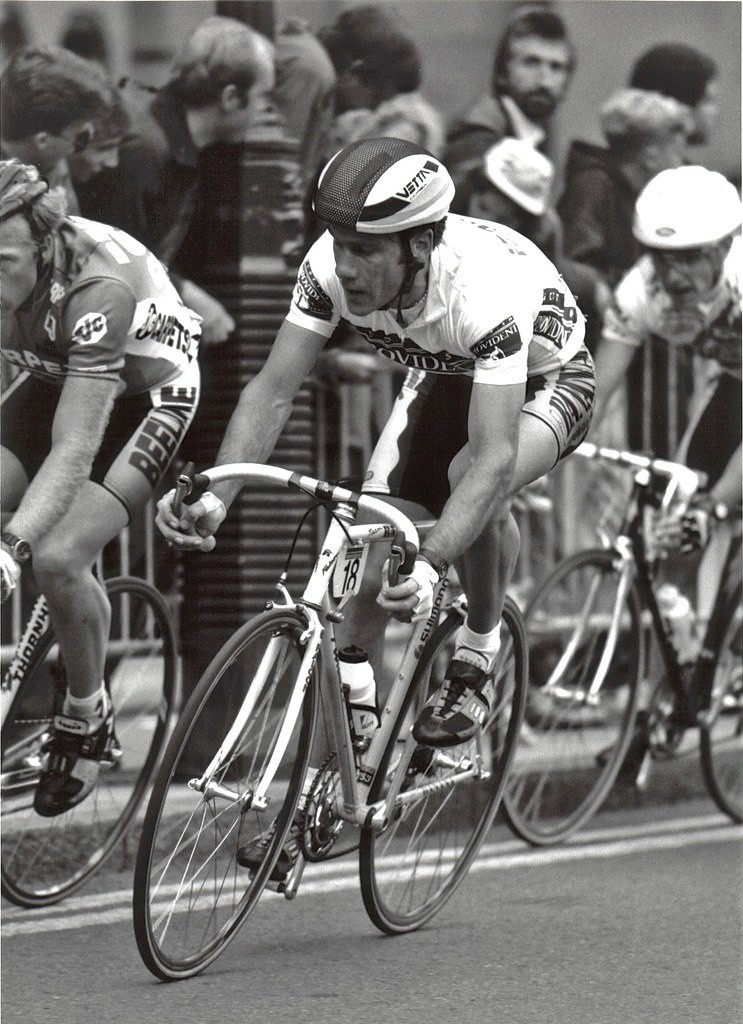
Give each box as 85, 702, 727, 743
2, 531, 185, 909
132, 459, 533, 983
498, 440, 743, 846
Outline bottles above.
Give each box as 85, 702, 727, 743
656, 589, 691, 652
338, 645, 377, 733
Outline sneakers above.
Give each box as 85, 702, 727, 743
237, 807, 313, 873
412, 646, 500, 747
33, 691, 115, 817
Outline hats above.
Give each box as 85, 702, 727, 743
484, 138, 554, 216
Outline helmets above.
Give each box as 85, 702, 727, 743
311, 137, 455, 234
0, 157, 48, 221
632, 165, 742, 248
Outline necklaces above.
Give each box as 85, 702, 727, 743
381, 290, 427, 310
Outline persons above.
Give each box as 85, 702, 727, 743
150, 137, 598, 877
0, 5, 743, 732
590, 166, 743, 793
0, 156, 203, 819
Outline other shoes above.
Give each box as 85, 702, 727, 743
596, 711, 651, 786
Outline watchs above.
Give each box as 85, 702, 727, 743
710, 498, 729, 521
420, 548, 449, 582
2, 530, 31, 566
